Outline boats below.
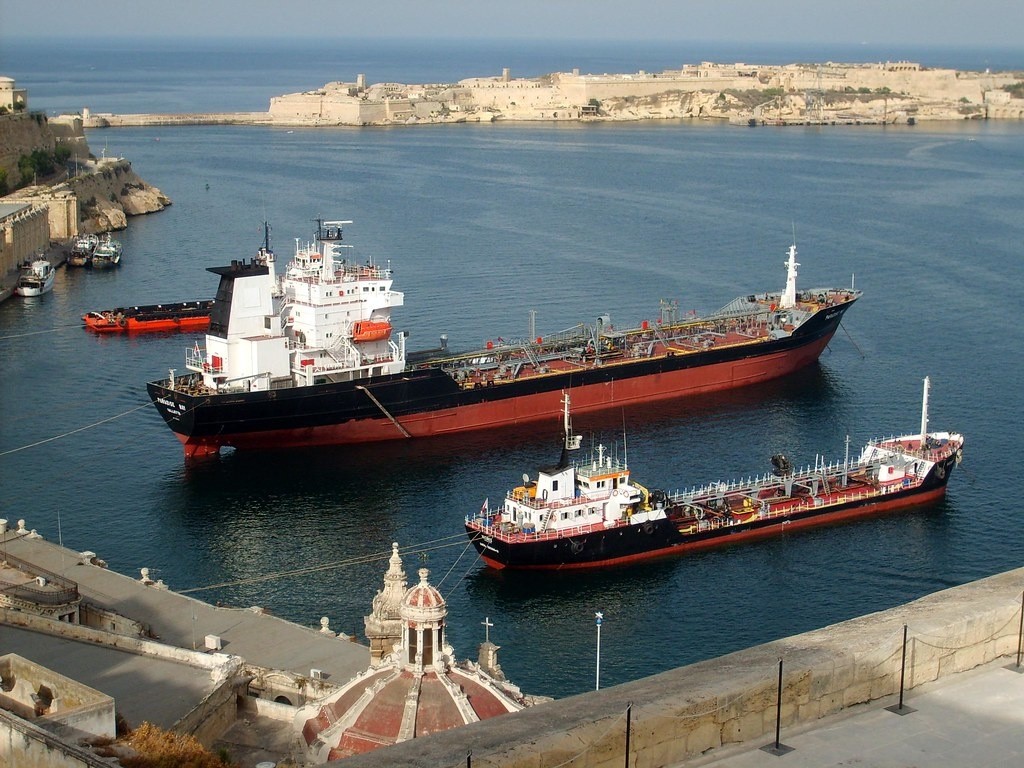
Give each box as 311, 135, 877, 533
464, 372, 965, 576
91, 232, 122, 268
68, 232, 100, 266
146, 217, 862, 460
16, 258, 56, 295
81, 301, 209, 335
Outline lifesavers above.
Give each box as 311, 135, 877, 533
118, 319, 127, 327
645, 525, 654, 535
575, 543, 584, 552
